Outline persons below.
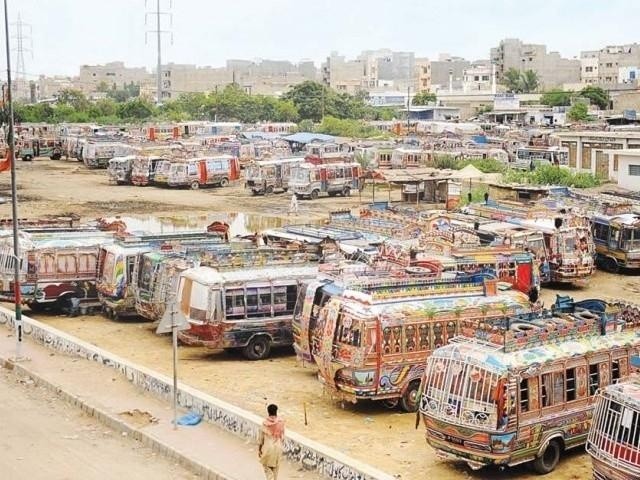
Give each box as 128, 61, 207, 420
409, 244, 422, 266
287, 192, 299, 217
577, 237, 593, 267
64, 294, 80, 317
527, 284, 539, 305
258, 404, 286, 480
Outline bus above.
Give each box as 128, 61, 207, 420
414, 298, 640, 477
582, 374, 640, 480
